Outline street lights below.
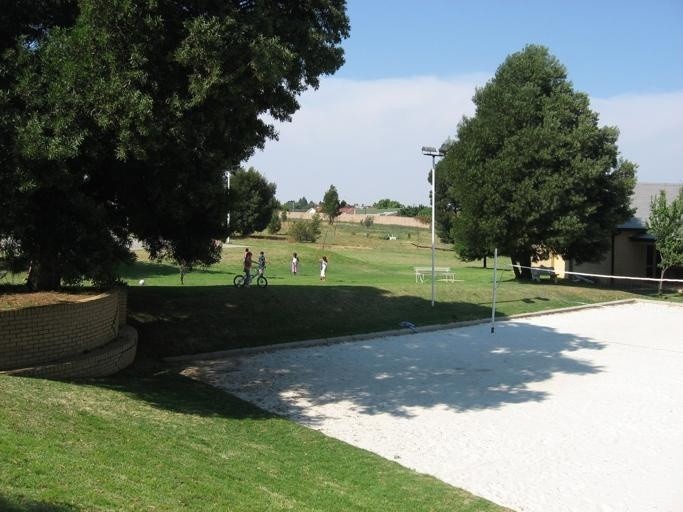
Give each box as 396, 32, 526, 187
225, 170, 233, 245
420, 145, 452, 310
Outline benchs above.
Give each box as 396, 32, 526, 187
414, 266, 456, 284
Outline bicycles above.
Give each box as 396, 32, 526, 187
233, 266, 267, 290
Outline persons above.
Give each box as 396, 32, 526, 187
257, 251, 269, 275
243, 248, 249, 262
243, 251, 261, 289
290, 252, 299, 275
319, 256, 328, 281
